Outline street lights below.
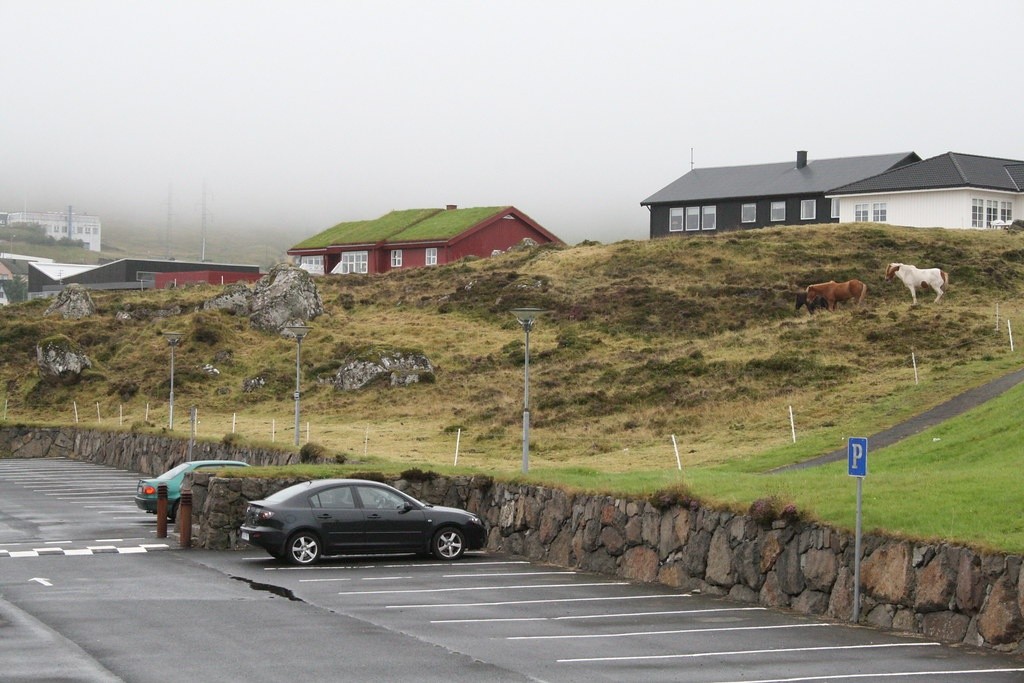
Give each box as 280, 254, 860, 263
163, 332, 185, 431
508, 307, 546, 474
285, 326, 314, 448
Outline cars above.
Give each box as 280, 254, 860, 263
134, 459, 254, 523
241, 479, 489, 561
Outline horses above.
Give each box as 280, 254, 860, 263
884, 263, 948, 303
795, 279, 868, 315
